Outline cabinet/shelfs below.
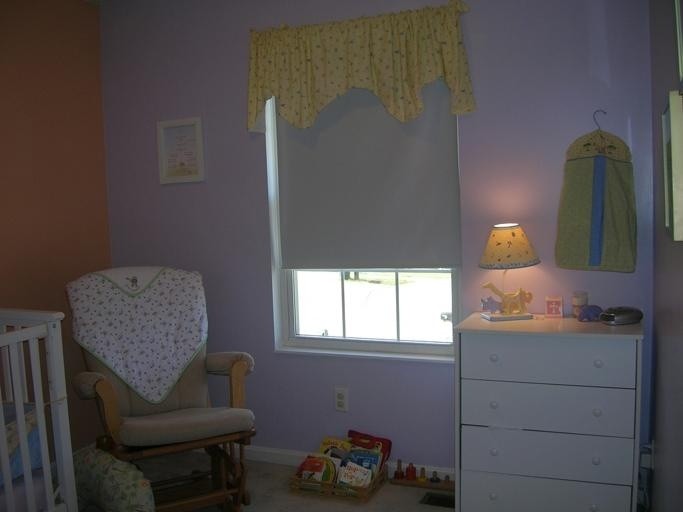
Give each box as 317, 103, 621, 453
451, 309, 649, 512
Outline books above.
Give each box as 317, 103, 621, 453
296, 429, 391, 496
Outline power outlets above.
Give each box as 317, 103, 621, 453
334, 388, 348, 412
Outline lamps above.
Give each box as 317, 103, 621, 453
474, 223, 542, 324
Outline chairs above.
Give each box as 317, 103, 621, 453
63, 263, 256, 510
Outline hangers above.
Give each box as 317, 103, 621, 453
565, 109, 632, 163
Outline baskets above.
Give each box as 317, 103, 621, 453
288, 464, 388, 502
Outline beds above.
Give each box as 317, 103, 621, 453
0, 305, 81, 511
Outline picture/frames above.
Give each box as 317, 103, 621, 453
156, 117, 205, 186
660, 90, 683, 242
